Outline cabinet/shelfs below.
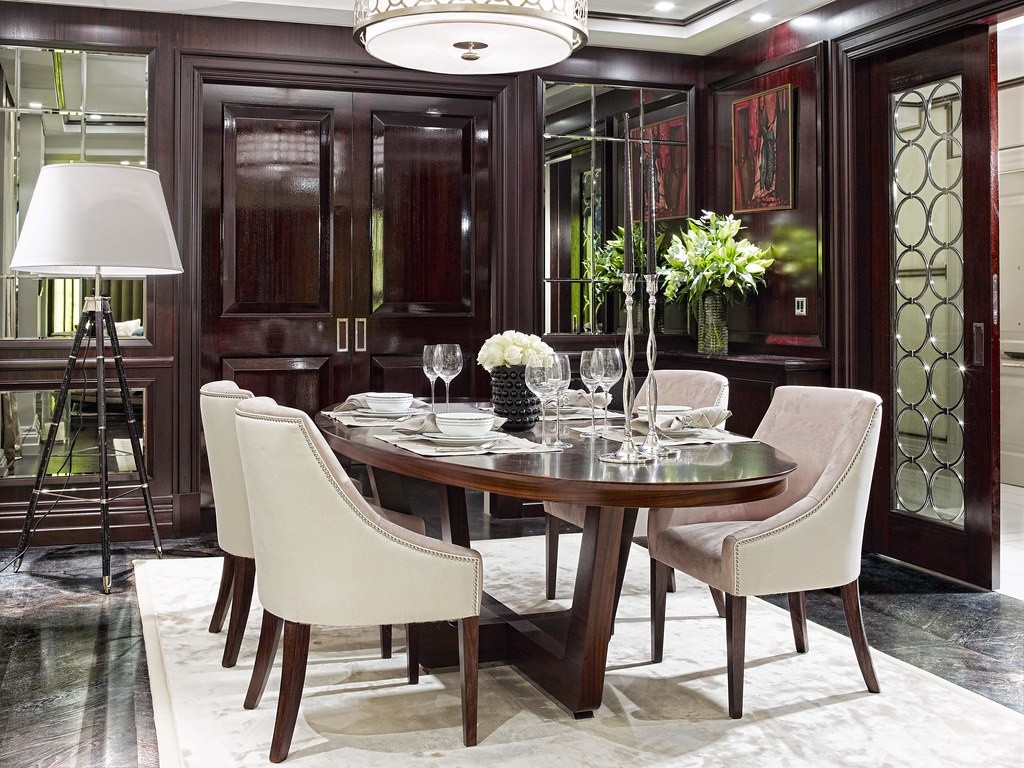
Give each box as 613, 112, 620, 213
657, 349, 829, 438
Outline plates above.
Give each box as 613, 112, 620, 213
421, 432, 503, 447
663, 429, 698, 437
540, 408, 580, 414
353, 408, 424, 418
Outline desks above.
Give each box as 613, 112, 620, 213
314, 395, 797, 717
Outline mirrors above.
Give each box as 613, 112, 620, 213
532, 71, 698, 344
0, 37, 159, 350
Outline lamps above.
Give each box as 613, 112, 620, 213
353, 0, 590, 76
9, 162, 184, 595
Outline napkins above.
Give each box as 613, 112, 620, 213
548, 388, 613, 409
630, 407, 732, 437
391, 412, 509, 436
333, 392, 430, 413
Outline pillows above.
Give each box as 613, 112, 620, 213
104, 319, 141, 337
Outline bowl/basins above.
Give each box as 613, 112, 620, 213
435, 413, 495, 436
638, 406, 692, 425
366, 392, 413, 411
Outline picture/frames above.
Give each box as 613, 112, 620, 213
630, 114, 687, 222
731, 83, 794, 215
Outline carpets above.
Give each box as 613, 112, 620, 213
113, 438, 143, 470
130, 531, 1024, 767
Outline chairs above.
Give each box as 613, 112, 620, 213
198, 379, 485, 763
541, 369, 885, 719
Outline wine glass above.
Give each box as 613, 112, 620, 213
423, 344, 439, 415
525, 354, 573, 449
580, 348, 623, 438
433, 344, 463, 413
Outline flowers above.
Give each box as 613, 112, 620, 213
654, 208, 774, 323
581, 223, 669, 313
477, 329, 553, 372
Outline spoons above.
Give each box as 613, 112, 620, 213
435, 439, 499, 451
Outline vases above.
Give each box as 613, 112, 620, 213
635, 282, 666, 336
696, 291, 729, 357
491, 364, 546, 431
